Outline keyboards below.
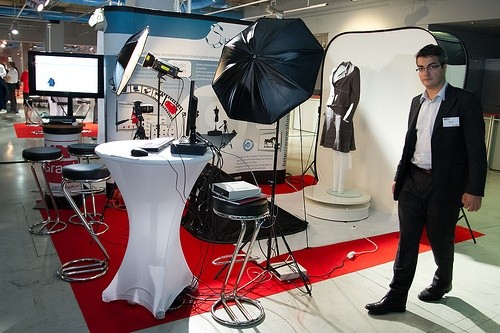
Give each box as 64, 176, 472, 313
140, 138, 175, 151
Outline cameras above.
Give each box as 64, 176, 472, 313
134, 101, 154, 116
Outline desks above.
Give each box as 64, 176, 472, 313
97, 140, 215, 319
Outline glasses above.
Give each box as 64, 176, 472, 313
416, 64, 442, 73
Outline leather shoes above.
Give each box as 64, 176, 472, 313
365, 295, 406, 315
418, 283, 452, 302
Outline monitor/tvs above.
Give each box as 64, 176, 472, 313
27, 50, 105, 98
186, 80, 201, 144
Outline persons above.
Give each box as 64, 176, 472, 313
364, 43, 488, 313
0, 61, 32, 124
320, 62, 360, 193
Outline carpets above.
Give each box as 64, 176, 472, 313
14, 122, 98, 139
37, 174, 486, 333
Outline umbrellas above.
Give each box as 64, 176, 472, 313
210, 17, 324, 125
115, 26, 149, 96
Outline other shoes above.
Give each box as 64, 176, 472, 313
0, 109, 8, 113
26, 121, 32, 124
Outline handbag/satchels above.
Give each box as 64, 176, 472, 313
17, 79, 21, 90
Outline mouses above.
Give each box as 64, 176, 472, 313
131, 149, 148, 156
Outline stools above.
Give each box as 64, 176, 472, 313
21, 147, 67, 234
26, 98, 90, 123
57, 164, 111, 281
210, 198, 272, 327
67, 143, 99, 224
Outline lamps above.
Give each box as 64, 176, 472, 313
25, 0, 50, 12
11, 22, 19, 34
266, 4, 284, 19
